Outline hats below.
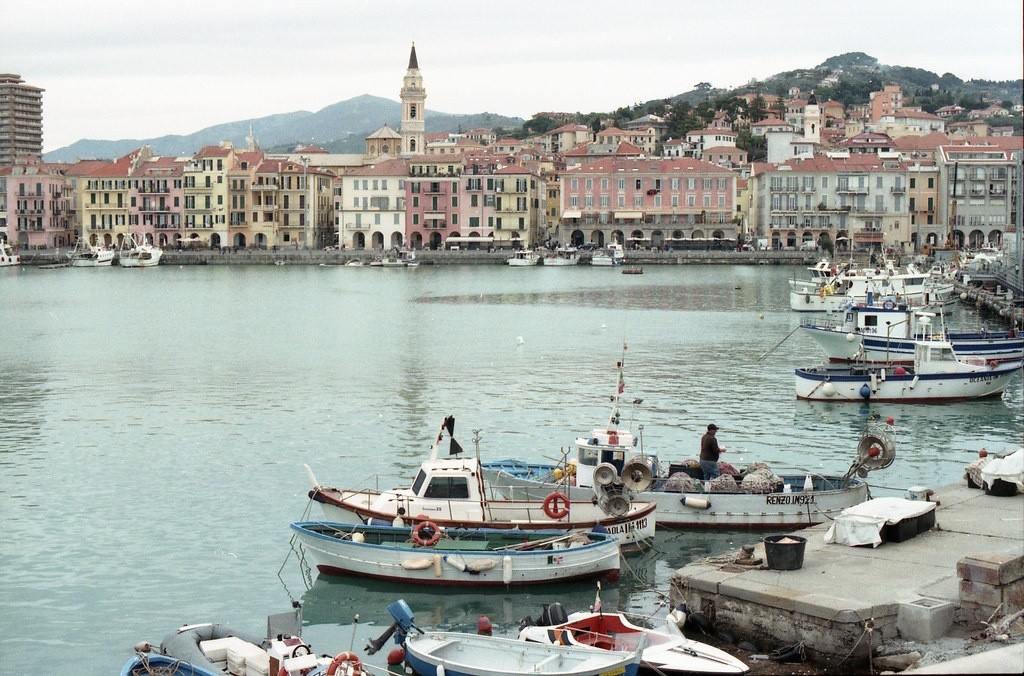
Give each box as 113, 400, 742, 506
708, 424, 719, 431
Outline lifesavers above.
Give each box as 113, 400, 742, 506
278, 665, 310, 676
737, 641, 758, 652
543, 492, 570, 519
413, 521, 440, 546
686, 613, 712, 635
715, 631, 733, 643
882, 299, 895, 311
769, 645, 801, 662
326, 651, 362, 676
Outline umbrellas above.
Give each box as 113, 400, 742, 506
627, 237, 651, 240
177, 236, 202, 242
494, 237, 523, 241
665, 237, 736, 241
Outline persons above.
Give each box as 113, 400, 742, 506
699, 424, 726, 480
706, 244, 710, 252
273, 245, 276, 253
218, 246, 238, 255
550, 244, 555, 253
738, 243, 742, 252
178, 242, 182, 254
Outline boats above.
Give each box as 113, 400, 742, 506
794, 239, 1024, 403
308, 415, 658, 556
588, 235, 627, 267
68, 237, 115, 267
119, 232, 164, 267
0, 239, 21, 267
343, 244, 421, 267
543, 242, 582, 266
506, 250, 541, 266
480, 335, 896, 527
387, 598, 650, 676
116, 599, 400, 676
622, 266, 644, 275
788, 234, 1024, 363
517, 580, 752, 676
290, 518, 622, 588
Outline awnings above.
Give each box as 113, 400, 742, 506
854, 236, 883, 242
615, 211, 642, 218
563, 211, 582, 218
